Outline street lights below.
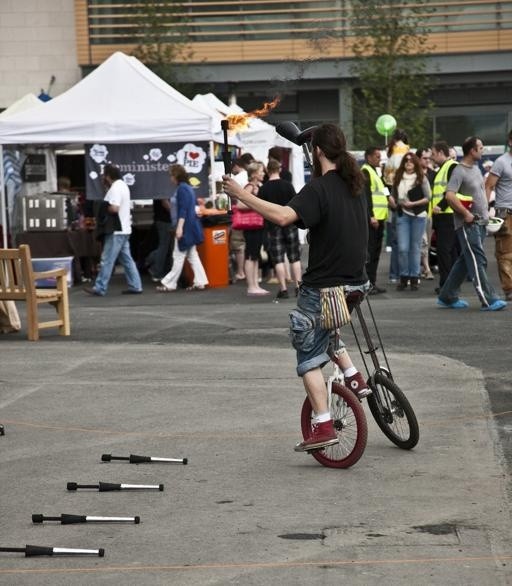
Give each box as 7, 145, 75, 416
374, 113, 399, 145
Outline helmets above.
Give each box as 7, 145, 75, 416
485, 216, 504, 233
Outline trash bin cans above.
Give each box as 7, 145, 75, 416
184, 226, 229, 288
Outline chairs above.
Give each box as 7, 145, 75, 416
1, 244, 72, 342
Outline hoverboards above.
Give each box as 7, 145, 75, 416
294, 293, 371, 470
342, 285, 421, 451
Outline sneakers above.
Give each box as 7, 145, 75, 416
335, 371, 373, 408
367, 270, 512, 301
292, 418, 340, 453
83, 272, 299, 299
479, 300, 507, 312
436, 294, 469, 310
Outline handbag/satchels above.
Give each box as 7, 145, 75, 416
317, 285, 352, 331
231, 183, 265, 230
407, 187, 429, 216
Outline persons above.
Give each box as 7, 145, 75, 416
83, 164, 144, 296
156, 164, 210, 292
223, 123, 375, 452
145, 199, 173, 282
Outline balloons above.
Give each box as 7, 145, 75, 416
376, 114, 398, 136
